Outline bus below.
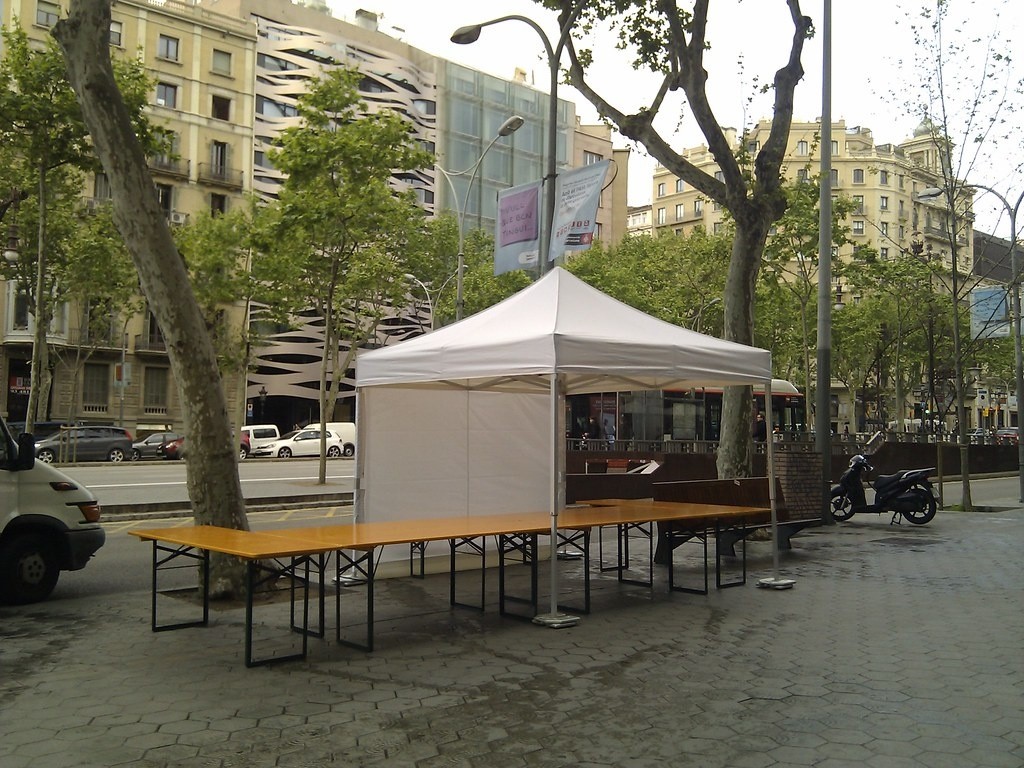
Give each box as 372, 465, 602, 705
567, 378, 803, 454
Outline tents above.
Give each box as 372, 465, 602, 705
352, 265, 779, 613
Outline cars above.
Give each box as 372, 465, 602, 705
126, 430, 185, 461
154, 429, 252, 460
253, 428, 344, 458
966, 424, 1020, 446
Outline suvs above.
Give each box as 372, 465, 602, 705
34, 424, 133, 464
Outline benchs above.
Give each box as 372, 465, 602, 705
652, 476, 822, 562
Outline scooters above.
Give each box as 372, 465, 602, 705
829, 451, 942, 527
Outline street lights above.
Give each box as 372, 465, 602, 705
403, 264, 469, 332
916, 183, 1024, 505
798, 270, 848, 443
985, 377, 1018, 428
449, 0, 592, 280
429, 115, 526, 321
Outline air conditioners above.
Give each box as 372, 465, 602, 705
171, 212, 185, 224
81, 197, 100, 215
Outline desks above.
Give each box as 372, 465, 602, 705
126, 500, 772, 668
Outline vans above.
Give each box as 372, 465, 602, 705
303, 421, 356, 457
0, 414, 106, 606
240, 423, 281, 456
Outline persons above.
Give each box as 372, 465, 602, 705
585, 416, 599, 439
603, 420, 616, 443
844, 426, 848, 436
752, 414, 767, 442
